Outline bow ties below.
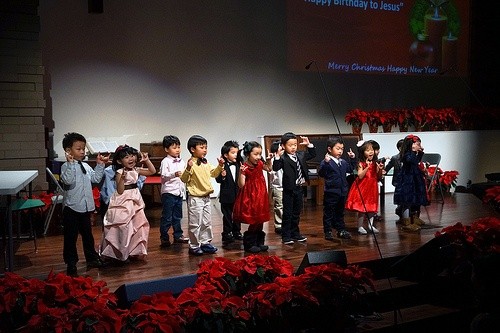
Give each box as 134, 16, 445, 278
172, 158, 181, 163
197, 158, 207, 166
226, 160, 237, 167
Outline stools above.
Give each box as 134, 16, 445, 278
144, 177, 162, 201
11, 199, 45, 255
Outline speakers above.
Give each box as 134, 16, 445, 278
393, 234, 461, 281
114, 273, 197, 309
295, 250, 348, 276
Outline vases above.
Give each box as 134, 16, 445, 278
444, 125, 450, 130
352, 126, 361, 136
400, 124, 408, 132
429, 126, 438, 130
383, 126, 391, 132
369, 125, 378, 133
415, 125, 424, 132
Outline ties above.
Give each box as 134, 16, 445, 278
292, 156, 303, 189
78, 160, 86, 174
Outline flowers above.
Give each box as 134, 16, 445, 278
428, 167, 500, 263
345, 105, 464, 128
0, 253, 378, 333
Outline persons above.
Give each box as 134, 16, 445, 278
180, 135, 225, 255
60, 132, 104, 275
318, 137, 356, 239
160, 135, 189, 249
273, 132, 317, 245
385, 135, 430, 231
344, 140, 386, 234
215, 140, 244, 242
231, 141, 276, 252
270, 141, 283, 234
99, 144, 156, 267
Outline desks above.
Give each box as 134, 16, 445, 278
0, 170, 39, 272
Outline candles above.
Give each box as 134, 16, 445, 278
424, 7, 449, 40
442, 32, 459, 70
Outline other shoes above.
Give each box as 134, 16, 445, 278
189, 246, 204, 256
200, 244, 218, 253
275, 228, 282, 234
66, 265, 77, 277
87, 255, 106, 268
173, 237, 190, 244
233, 231, 244, 240
373, 213, 384, 222
325, 230, 335, 240
281, 236, 295, 245
161, 243, 177, 250
291, 234, 307, 242
221, 231, 236, 241
336, 229, 352, 239
395, 206, 402, 218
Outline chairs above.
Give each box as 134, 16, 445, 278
44, 166, 64, 234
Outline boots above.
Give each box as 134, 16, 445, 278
243, 231, 261, 253
410, 213, 426, 226
367, 216, 379, 233
401, 217, 421, 232
258, 231, 269, 251
357, 217, 367, 235
